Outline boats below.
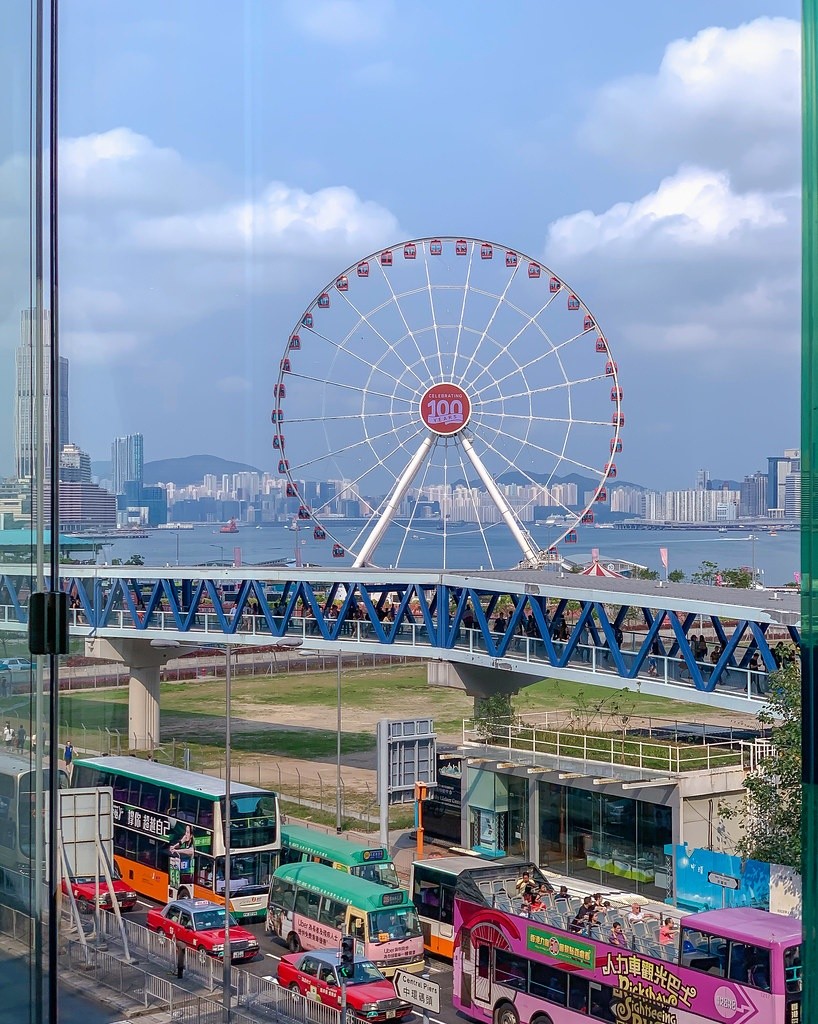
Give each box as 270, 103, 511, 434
218, 520, 240, 534
289, 522, 301, 531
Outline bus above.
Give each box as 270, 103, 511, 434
279, 823, 400, 892
452, 861, 805, 1024
265, 862, 425, 977
0, 750, 71, 880
409, 855, 700, 964
68, 755, 289, 921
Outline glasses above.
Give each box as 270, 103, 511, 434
672, 922, 674, 924
536, 896, 541, 900
636, 907, 640, 909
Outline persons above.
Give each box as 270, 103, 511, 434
646, 634, 797, 695
169, 826, 195, 874
169, 911, 226, 931
102, 752, 158, 763
63, 741, 80, 770
449, 604, 623, 663
3, 720, 37, 755
68, 587, 406, 638
514, 871, 680, 964
336, 911, 346, 930
714, 571, 723, 587
325, 970, 335, 982
742, 944, 802, 994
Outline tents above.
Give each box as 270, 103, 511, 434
573, 560, 629, 580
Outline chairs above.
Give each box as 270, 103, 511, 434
424, 888, 440, 907
478, 872, 770, 992
273, 889, 339, 928
114, 788, 214, 869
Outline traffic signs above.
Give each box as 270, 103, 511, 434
391, 968, 440, 1014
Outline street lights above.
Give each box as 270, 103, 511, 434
534, 523, 563, 570
284, 525, 311, 567
149, 636, 304, 1023
170, 531, 179, 566
209, 544, 224, 567
298, 650, 342, 836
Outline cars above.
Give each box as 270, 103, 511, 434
61, 869, 139, 914
144, 897, 260, 966
278, 947, 413, 1023
1, 657, 46, 672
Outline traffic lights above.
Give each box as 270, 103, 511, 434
338, 937, 354, 978
